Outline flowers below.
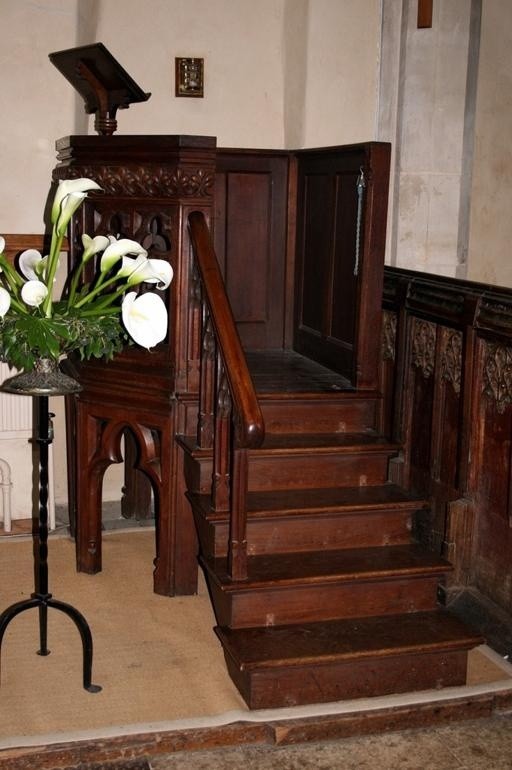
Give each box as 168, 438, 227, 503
1, 175, 177, 375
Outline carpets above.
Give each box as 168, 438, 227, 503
0, 527, 512, 753
